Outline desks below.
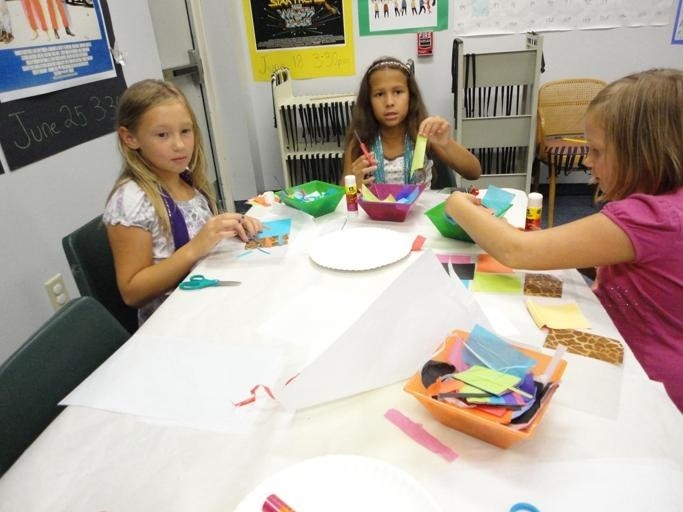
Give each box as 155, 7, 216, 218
1, 195, 682, 511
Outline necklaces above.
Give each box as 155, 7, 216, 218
379, 128, 403, 139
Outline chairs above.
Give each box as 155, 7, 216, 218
0, 215, 139, 479
535, 77, 608, 230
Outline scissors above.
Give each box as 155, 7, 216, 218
179, 274, 241, 290
353, 130, 372, 167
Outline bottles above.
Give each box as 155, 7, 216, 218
526, 192, 543, 231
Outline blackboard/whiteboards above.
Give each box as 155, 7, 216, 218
0, 0, 129, 173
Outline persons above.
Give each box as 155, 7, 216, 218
99, 78, 264, 326
444, 68, 683, 412
0, 0, 75, 45
338, 57, 481, 192
